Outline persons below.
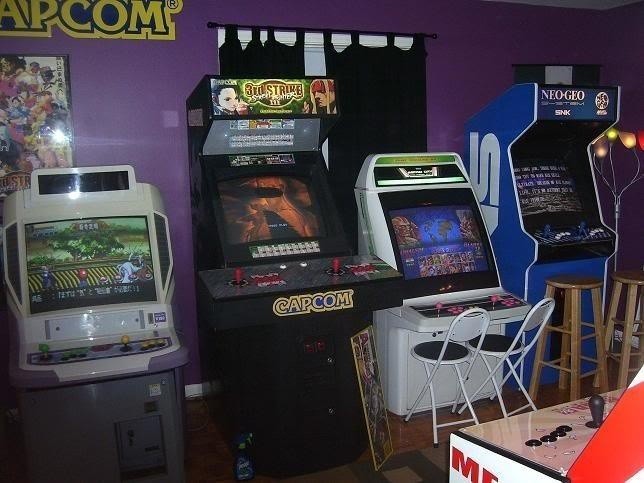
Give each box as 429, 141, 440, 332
302, 78, 337, 114
209, 78, 252, 116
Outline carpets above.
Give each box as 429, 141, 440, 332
280, 443, 449, 483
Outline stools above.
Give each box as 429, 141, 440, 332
593, 269, 644, 389
529, 275, 610, 405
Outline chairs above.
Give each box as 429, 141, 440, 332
403, 308, 491, 448
456, 297, 556, 417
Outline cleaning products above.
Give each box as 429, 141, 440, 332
235, 431, 255, 481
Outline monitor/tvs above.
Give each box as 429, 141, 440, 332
24, 215, 158, 314
216, 174, 328, 246
513, 164, 583, 217
378, 189, 500, 298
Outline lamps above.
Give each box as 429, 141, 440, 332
592, 127, 644, 271
